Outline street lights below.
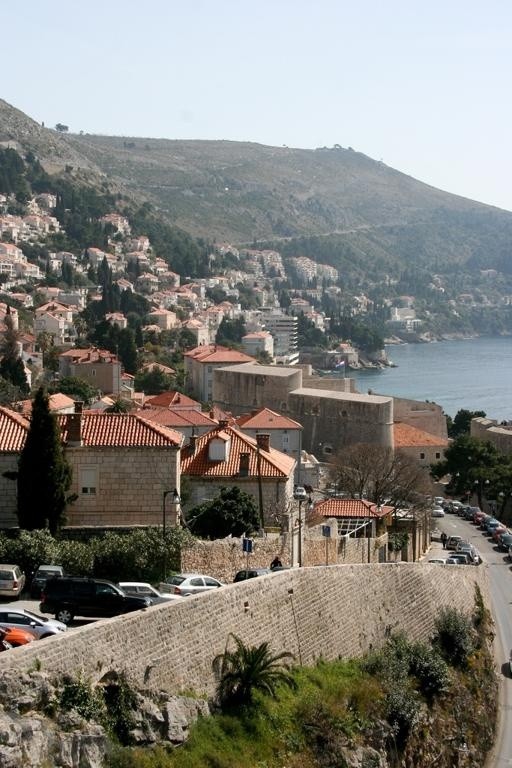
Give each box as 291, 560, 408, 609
298, 498, 313, 567
162, 488, 181, 580
368, 504, 382, 563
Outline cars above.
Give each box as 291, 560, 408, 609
426, 495, 512, 564
158, 574, 226, 596
31, 565, 64, 593
104, 581, 185, 606
0, 606, 67, 646
233, 568, 273, 582
0, 564, 25, 597
295, 487, 306, 499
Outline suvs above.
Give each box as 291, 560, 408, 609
38, 575, 153, 624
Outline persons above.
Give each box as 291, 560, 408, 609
269, 556, 283, 570
440, 531, 447, 548
